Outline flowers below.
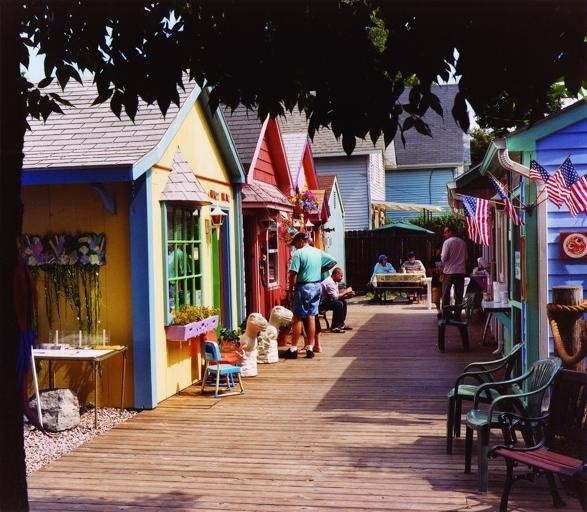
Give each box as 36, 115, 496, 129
290, 190, 323, 221
19, 232, 106, 333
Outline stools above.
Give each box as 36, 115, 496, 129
313, 309, 331, 332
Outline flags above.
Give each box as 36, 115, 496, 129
488, 152, 586, 227
461, 195, 491, 248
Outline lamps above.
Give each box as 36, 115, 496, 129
204, 206, 225, 246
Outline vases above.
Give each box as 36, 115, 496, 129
302, 221, 312, 235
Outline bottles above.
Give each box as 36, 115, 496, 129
497, 281, 504, 292
482, 290, 487, 301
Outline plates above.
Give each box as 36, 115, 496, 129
562, 233, 586, 258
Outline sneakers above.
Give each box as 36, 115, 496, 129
279, 344, 322, 358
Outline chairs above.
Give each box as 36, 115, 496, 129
486, 365, 585, 512
436, 294, 476, 354
445, 340, 524, 455
464, 355, 563, 497
197, 339, 246, 400
202, 336, 241, 387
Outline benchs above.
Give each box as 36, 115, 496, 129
372, 270, 432, 308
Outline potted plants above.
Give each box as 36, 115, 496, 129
166, 304, 220, 342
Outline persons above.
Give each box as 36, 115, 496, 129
319, 266, 355, 335
366, 223, 470, 324
278, 232, 337, 360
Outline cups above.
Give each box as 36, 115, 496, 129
499, 291, 508, 304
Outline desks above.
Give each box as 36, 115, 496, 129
30, 343, 129, 429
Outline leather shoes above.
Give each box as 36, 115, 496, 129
340, 325, 353, 330
331, 329, 345, 333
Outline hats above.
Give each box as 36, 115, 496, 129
290, 233, 308, 245
379, 254, 388, 260
407, 252, 415, 256
